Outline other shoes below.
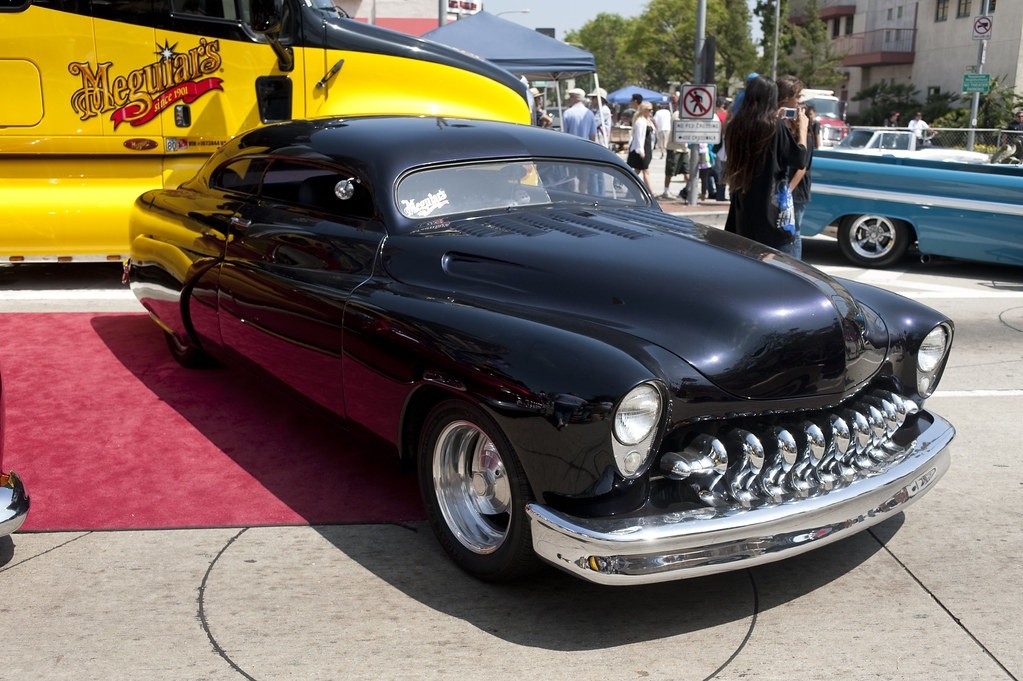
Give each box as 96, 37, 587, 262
663, 192, 677, 199
652, 193, 662, 200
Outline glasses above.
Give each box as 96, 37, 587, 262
646, 109, 653, 112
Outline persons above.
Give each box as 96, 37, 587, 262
626, 93, 733, 202
908, 111, 938, 150
1006, 112, 1023, 160
883, 112, 900, 127
529, 87, 611, 196
723, 73, 819, 260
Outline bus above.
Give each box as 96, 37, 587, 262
1, 1, 534, 297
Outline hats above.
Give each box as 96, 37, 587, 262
889, 111, 900, 117
529, 88, 544, 98
567, 88, 585, 100
587, 87, 609, 105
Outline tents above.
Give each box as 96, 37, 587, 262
418, 10, 618, 199
607, 85, 663, 124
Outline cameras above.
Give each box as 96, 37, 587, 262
784, 108, 799, 119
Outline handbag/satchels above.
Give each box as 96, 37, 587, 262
769, 122, 796, 238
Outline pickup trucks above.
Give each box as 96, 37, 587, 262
795, 151, 1023, 270
833, 127, 991, 164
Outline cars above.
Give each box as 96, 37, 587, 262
120, 116, 957, 586
546, 107, 569, 127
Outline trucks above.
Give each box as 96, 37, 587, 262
798, 88, 849, 148
